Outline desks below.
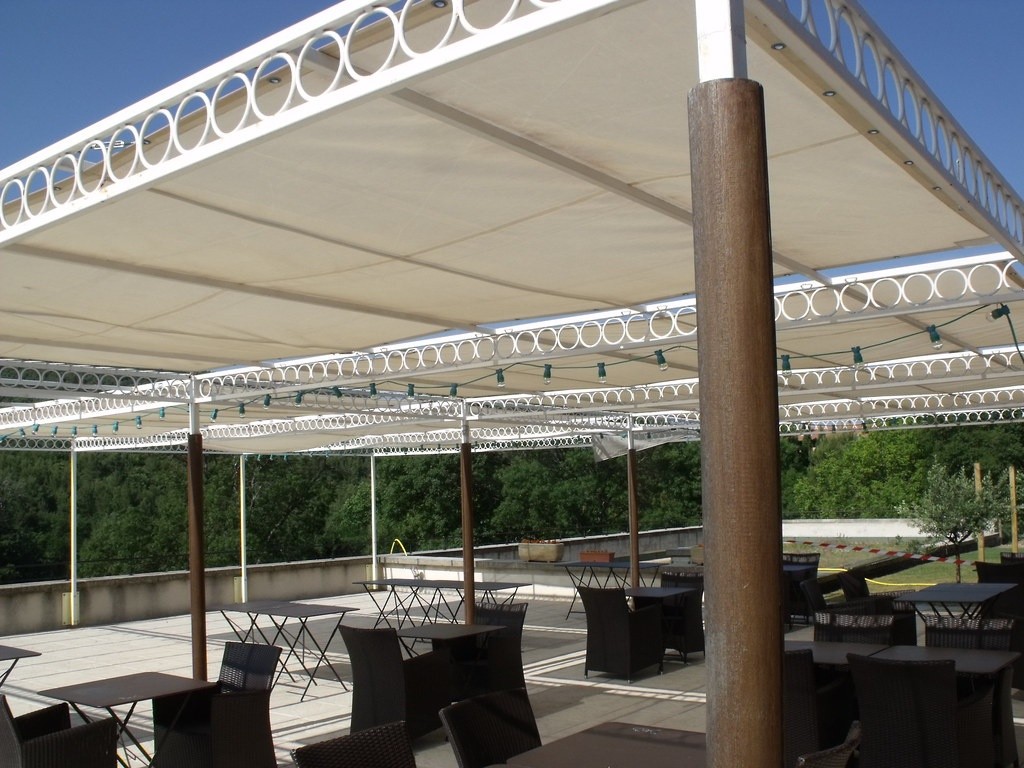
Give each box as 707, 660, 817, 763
782, 564, 817, 625
552, 561, 671, 620
486, 721, 709, 768
0, 643, 43, 689
37, 672, 219, 768
843, 644, 1021, 768
205, 600, 361, 701
352, 579, 532, 650
396, 623, 508, 742
782, 639, 890, 671
892, 582, 1018, 623
623, 587, 699, 661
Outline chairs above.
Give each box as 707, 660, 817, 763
0, 552, 1024, 768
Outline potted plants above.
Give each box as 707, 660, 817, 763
518, 538, 564, 563
690, 545, 704, 565
577, 552, 615, 563
